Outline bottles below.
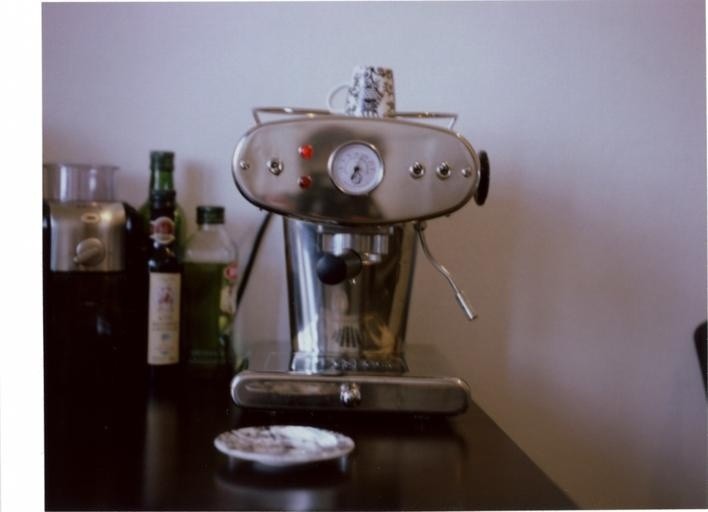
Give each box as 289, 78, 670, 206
137, 151, 239, 386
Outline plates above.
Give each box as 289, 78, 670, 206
213, 424, 356, 468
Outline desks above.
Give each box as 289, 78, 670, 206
46, 334, 583, 509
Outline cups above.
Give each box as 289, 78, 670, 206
43, 162, 119, 202
